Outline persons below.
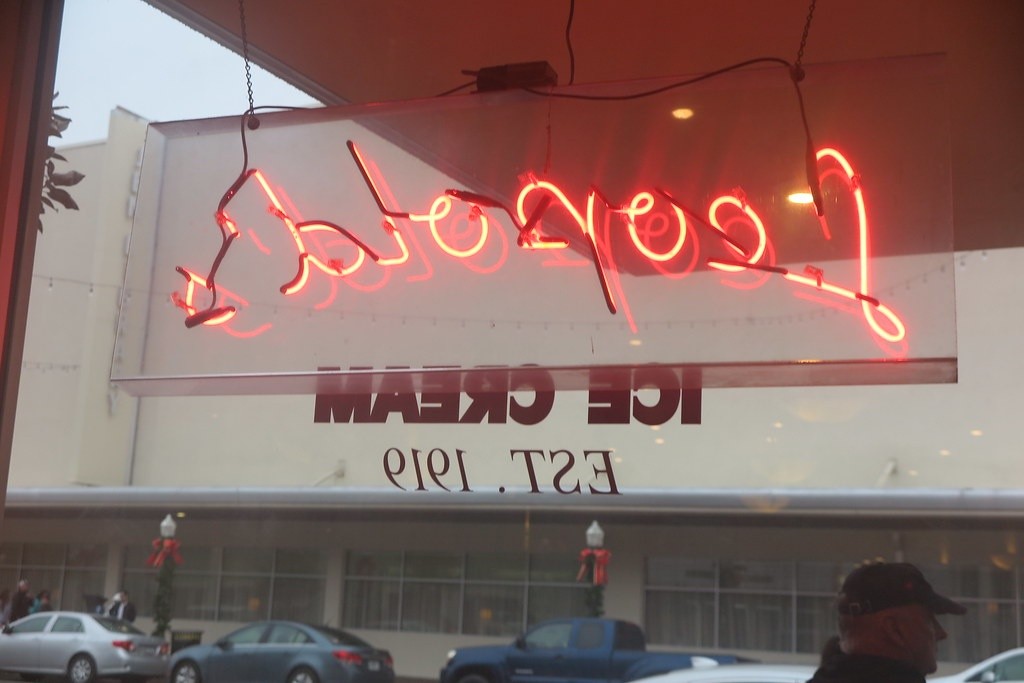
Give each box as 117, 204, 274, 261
5, 581, 50, 623
807, 563, 965, 683
109, 590, 136, 622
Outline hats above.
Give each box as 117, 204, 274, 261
837, 562, 967, 616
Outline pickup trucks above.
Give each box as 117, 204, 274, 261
440, 616, 761, 683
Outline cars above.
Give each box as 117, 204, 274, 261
165, 617, 393, 683
0, 610, 169, 683
926, 646, 1024, 683
